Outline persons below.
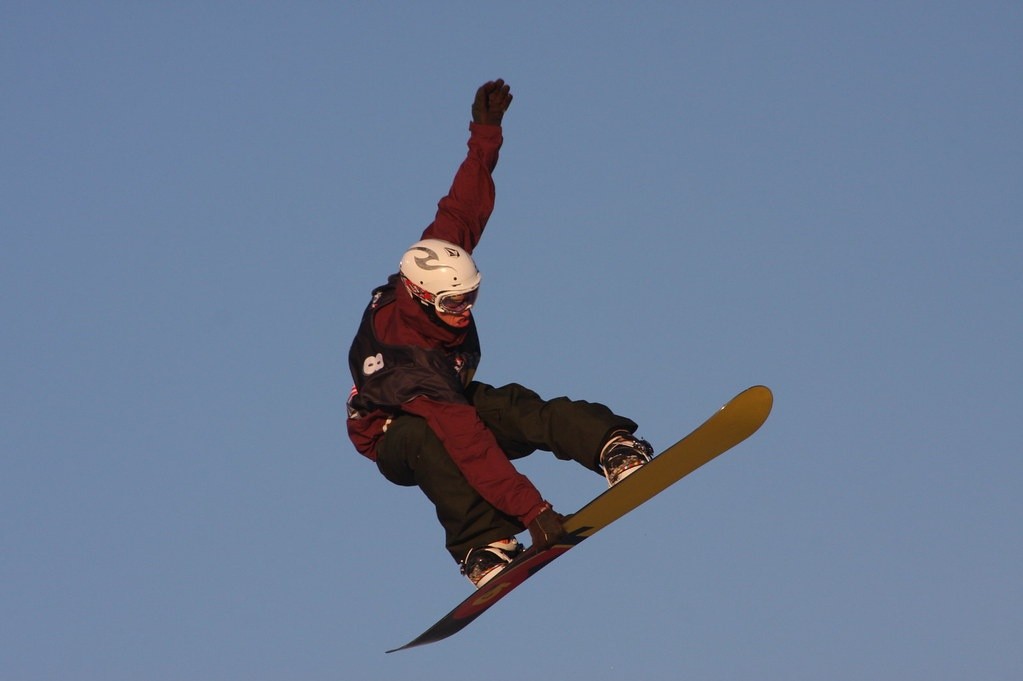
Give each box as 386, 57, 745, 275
347, 79, 654, 590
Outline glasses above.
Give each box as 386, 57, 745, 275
434, 286, 479, 311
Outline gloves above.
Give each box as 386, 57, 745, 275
527, 508, 575, 549
471, 78, 513, 127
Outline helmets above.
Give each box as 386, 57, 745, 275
399, 239, 482, 309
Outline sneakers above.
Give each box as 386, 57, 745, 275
459, 536, 527, 588
597, 429, 653, 487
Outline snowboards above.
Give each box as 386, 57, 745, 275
381, 385, 774, 653
